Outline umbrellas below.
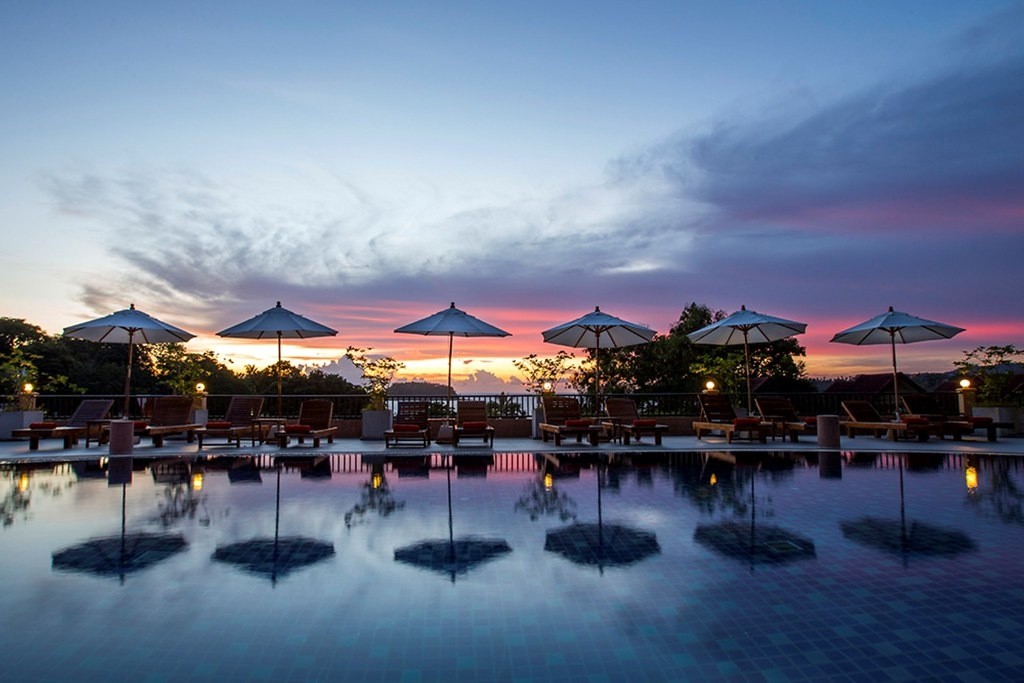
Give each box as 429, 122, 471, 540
61, 303, 198, 418
50, 484, 189, 587
839, 456, 977, 569
828, 306, 967, 420
392, 470, 514, 583
393, 300, 514, 426
543, 456, 663, 576
209, 470, 337, 585
214, 299, 339, 424
685, 303, 808, 416
693, 464, 819, 567
540, 305, 658, 423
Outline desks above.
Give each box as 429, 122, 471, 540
427, 418, 455, 444
593, 417, 621, 446
251, 418, 286, 447
85, 419, 114, 448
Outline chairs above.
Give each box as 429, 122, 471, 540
359, 454, 433, 482
193, 396, 265, 450
692, 393, 768, 446
452, 454, 495, 479
277, 457, 333, 481
546, 456, 580, 478
384, 402, 432, 447
454, 400, 494, 449
538, 396, 607, 448
86, 394, 192, 448
274, 399, 338, 450
12, 399, 114, 450
902, 396, 1014, 441
755, 397, 818, 444
152, 458, 190, 485
224, 456, 263, 485
841, 401, 938, 442
603, 398, 669, 445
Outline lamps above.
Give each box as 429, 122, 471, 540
959, 379, 973, 389
703, 380, 717, 390
193, 383, 210, 409
372, 473, 383, 488
545, 474, 555, 489
18, 472, 29, 493
18, 384, 39, 410
966, 466, 981, 494
193, 474, 205, 491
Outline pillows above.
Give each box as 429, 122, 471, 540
734, 418, 759, 426
29, 422, 57, 429
806, 417, 818, 425
392, 423, 419, 432
634, 420, 657, 427
565, 418, 593, 427
902, 415, 931, 424
205, 422, 231, 429
136, 421, 147, 429
965, 415, 993, 424
462, 421, 486, 430
285, 425, 309, 434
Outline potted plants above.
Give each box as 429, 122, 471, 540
0, 350, 43, 440
514, 351, 575, 439
345, 347, 405, 440
